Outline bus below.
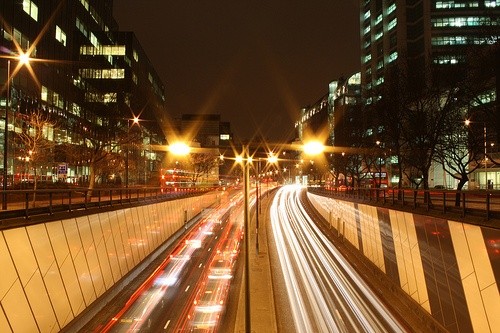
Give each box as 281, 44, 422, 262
357, 167, 390, 197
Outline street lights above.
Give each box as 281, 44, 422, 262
2, 51, 29, 210
247, 157, 260, 255
258, 158, 263, 213
125, 117, 139, 199
234, 155, 250, 332
464, 119, 488, 189
